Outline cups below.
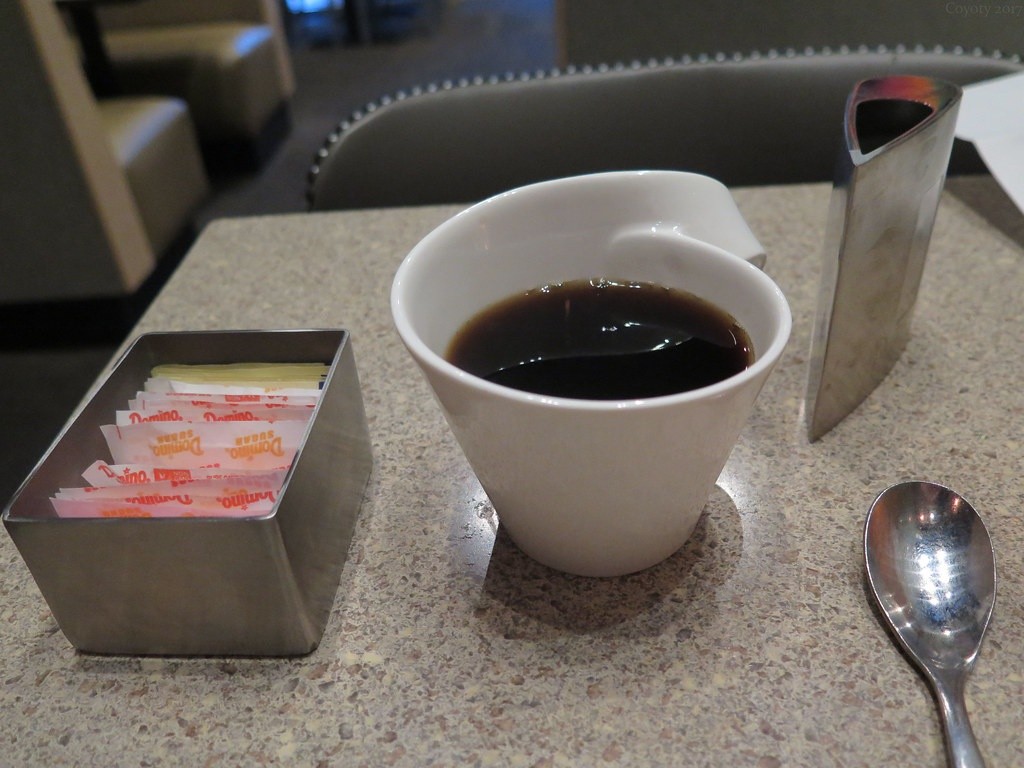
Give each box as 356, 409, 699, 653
390, 170, 792, 578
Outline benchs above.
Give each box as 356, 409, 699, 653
92, 0, 291, 145
67, 95, 209, 261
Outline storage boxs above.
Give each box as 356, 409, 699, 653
4, 328, 374, 658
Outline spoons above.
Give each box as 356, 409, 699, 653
864, 480, 995, 767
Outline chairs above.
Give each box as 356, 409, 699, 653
303, 45, 1023, 212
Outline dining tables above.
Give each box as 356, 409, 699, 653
0, 178, 1023, 768
